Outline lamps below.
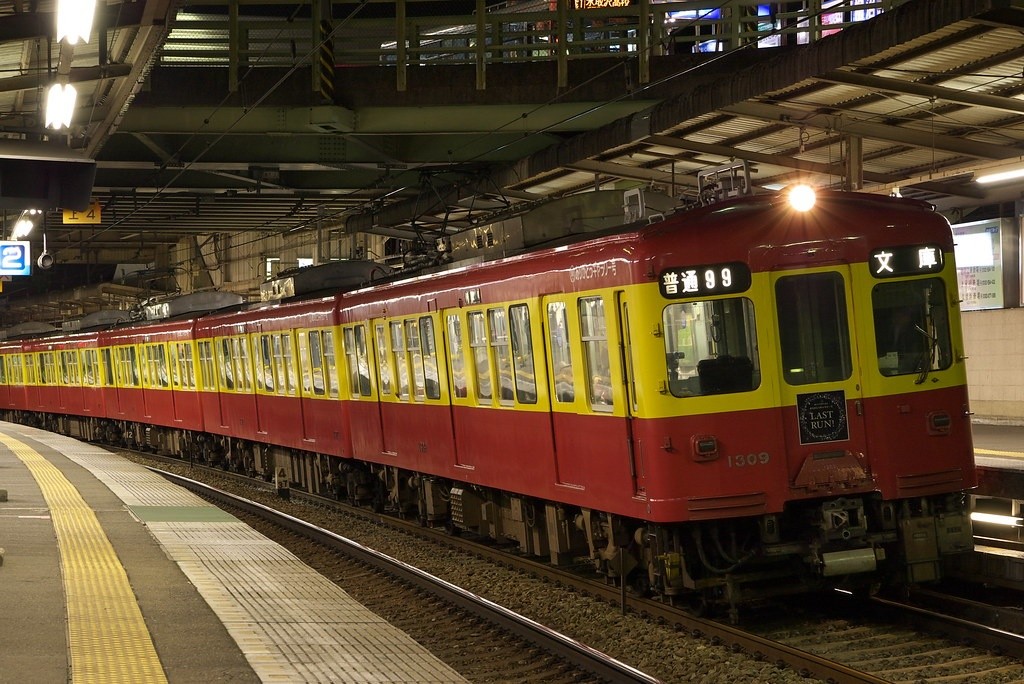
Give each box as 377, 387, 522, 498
44, 74, 76, 129
889, 188, 902, 197
13, 216, 33, 237
973, 161, 1024, 184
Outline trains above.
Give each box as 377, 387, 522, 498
0, 179, 980, 616
